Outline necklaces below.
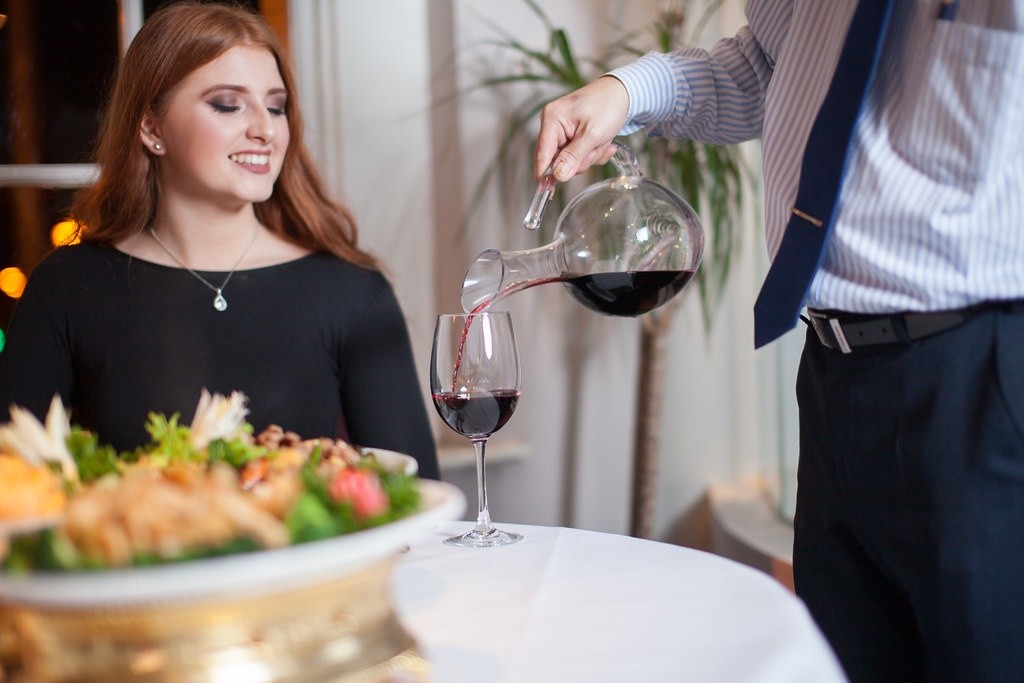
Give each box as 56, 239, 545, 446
150, 226, 258, 311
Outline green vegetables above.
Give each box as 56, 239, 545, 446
0, 409, 420, 580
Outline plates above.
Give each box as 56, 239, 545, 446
0, 474, 464, 610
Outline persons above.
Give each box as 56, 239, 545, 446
0, 5, 441, 480
535, 0, 1024, 683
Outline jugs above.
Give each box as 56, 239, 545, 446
460, 141, 705, 314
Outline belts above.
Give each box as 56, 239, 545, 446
807, 307, 966, 354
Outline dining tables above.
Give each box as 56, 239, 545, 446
398, 519, 848, 682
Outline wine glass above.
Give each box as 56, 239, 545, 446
430, 312, 522, 548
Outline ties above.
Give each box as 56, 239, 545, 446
753, 0, 894, 350
